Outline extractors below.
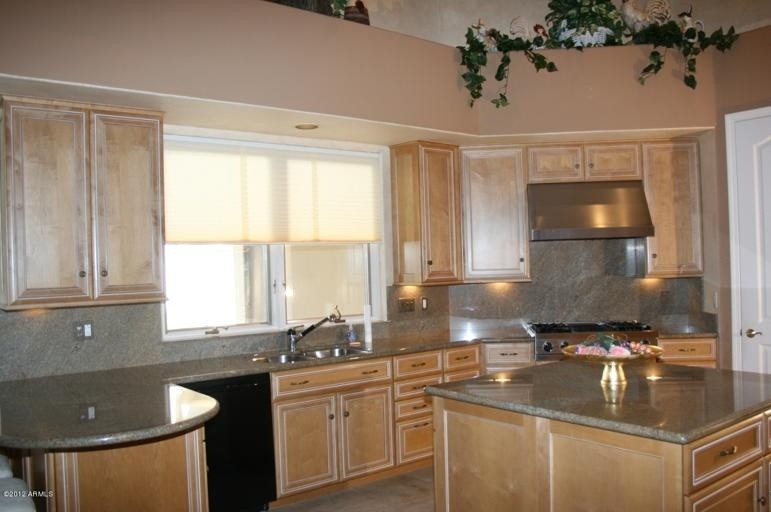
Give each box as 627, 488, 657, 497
526, 180, 656, 246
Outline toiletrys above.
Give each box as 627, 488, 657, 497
343, 321, 358, 343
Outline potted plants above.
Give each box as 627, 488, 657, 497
457, 1, 738, 110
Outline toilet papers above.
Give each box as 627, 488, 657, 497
364, 304, 372, 349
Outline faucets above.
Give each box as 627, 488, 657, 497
286, 314, 336, 351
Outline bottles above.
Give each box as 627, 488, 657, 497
346, 323, 359, 342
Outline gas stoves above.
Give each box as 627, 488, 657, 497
521, 320, 659, 355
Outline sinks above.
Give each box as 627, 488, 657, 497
249, 353, 315, 364
295, 346, 374, 358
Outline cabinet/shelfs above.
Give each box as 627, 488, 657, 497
527, 140, 643, 184
272, 356, 396, 504
393, 142, 462, 290
655, 339, 717, 367
1, 93, 169, 315
636, 139, 704, 277
392, 344, 484, 469
484, 338, 536, 376
460, 143, 532, 283
541, 407, 771, 512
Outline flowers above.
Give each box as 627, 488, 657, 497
567, 334, 654, 358
